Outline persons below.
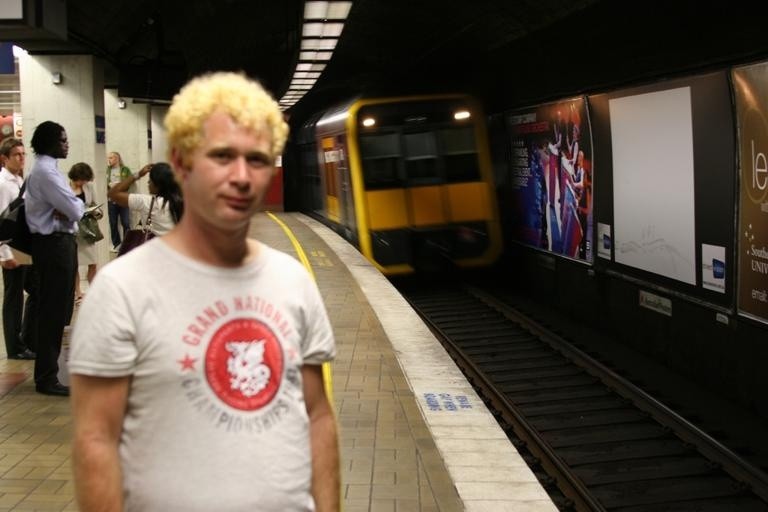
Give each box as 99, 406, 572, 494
107, 161, 182, 244
0, 137, 37, 362
24, 120, 87, 396
67, 162, 103, 303
105, 152, 135, 254
65, 71, 343, 512
530, 99, 591, 261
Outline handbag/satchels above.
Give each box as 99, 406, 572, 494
78, 214, 105, 245
0, 174, 38, 256
114, 195, 159, 260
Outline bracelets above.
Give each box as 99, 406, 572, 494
132, 172, 139, 180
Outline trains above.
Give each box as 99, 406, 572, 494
282, 91, 504, 278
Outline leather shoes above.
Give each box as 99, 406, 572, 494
8, 346, 38, 361
36, 380, 72, 396
